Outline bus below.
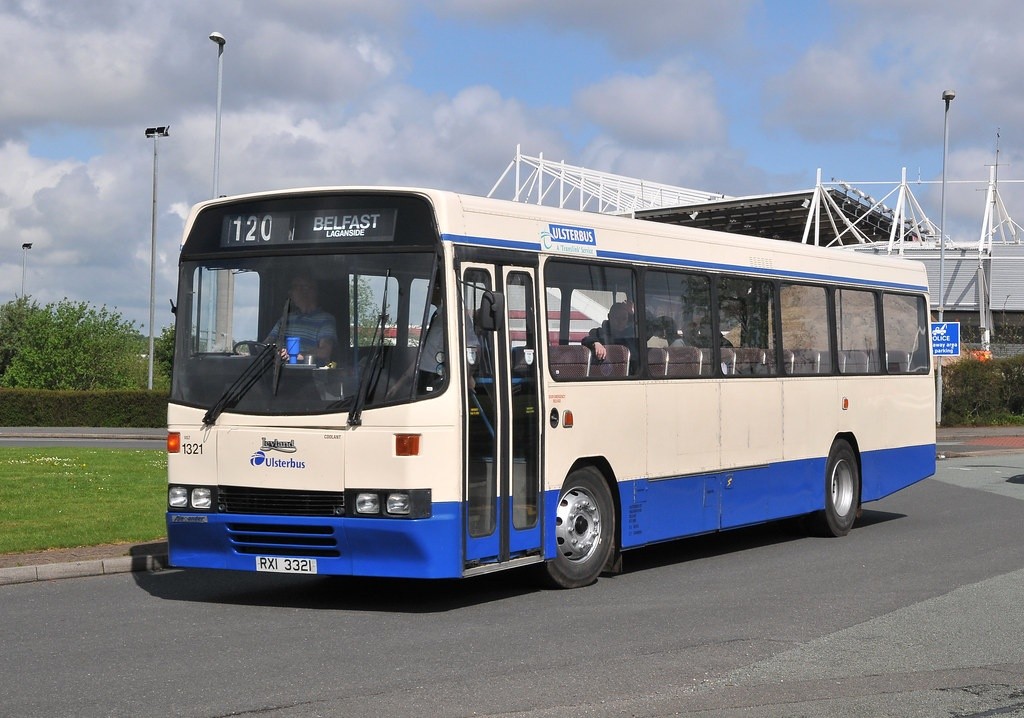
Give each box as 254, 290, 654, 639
165, 184, 937, 588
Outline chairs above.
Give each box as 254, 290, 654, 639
514, 346, 911, 378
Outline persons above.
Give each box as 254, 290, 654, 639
255, 275, 338, 363
657, 315, 734, 348
581, 300, 666, 361
417, 277, 481, 396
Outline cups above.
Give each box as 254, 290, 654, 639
287, 338, 300, 363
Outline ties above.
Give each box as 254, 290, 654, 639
424, 311, 437, 347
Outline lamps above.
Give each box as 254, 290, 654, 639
689, 212, 698, 220
801, 199, 811, 208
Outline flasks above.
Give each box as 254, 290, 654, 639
304, 354, 316, 365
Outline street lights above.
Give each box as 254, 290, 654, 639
208, 30, 228, 200
935, 89, 956, 425
